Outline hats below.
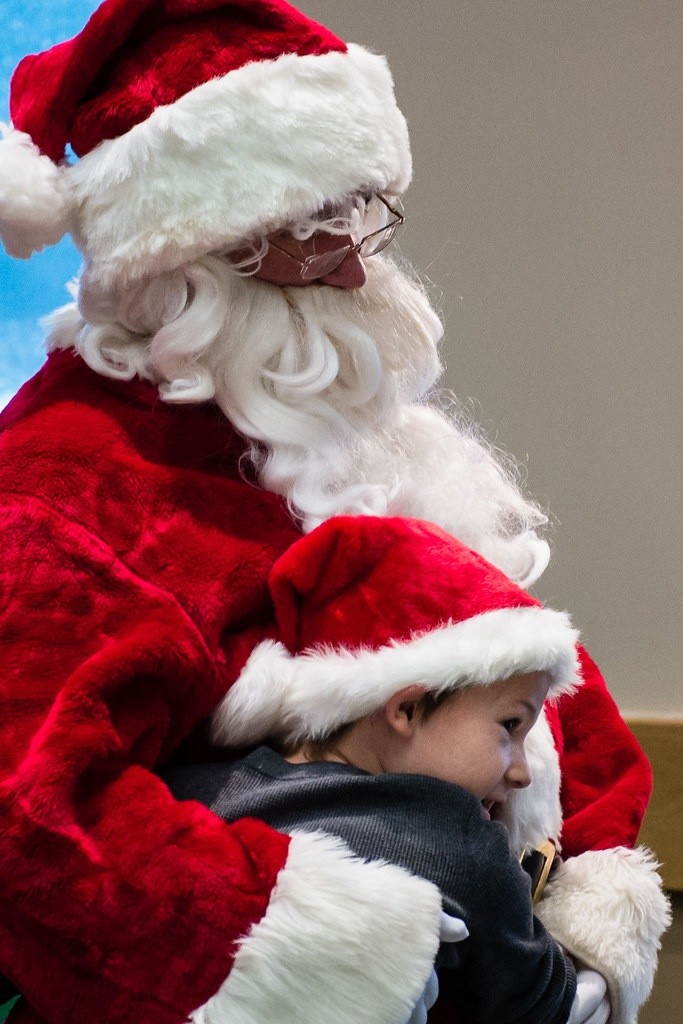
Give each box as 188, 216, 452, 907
210, 514, 584, 747
0, 0, 414, 294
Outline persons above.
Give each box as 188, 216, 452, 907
0, 0, 674, 1023
167, 511, 580, 1024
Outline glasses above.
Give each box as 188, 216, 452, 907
266, 191, 405, 281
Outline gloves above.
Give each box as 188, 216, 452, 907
565, 969, 611, 1024
406, 909, 469, 1024
